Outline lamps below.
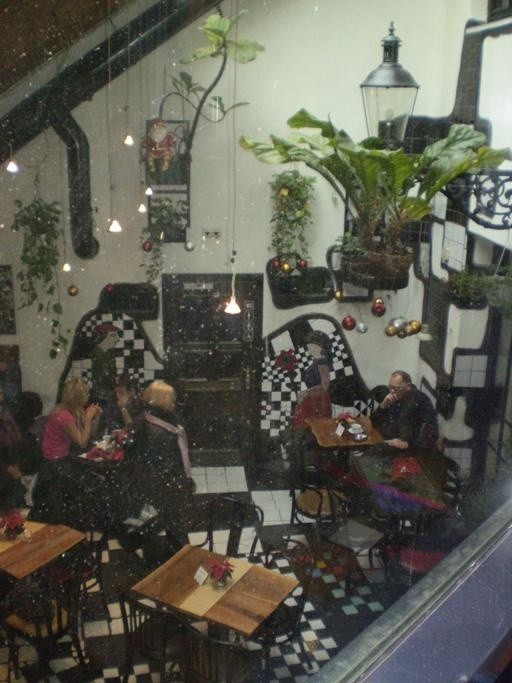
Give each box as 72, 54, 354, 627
359, 17, 417, 151
105, 0, 155, 233
5, 131, 19, 173
223, 32, 242, 316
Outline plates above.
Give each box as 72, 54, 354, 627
348, 426, 365, 435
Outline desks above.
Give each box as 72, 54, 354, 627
130, 544, 303, 671
3, 507, 88, 580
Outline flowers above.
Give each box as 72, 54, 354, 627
207, 556, 232, 581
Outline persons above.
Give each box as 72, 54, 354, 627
1, 371, 198, 623
368, 369, 442, 459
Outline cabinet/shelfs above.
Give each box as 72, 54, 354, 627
158, 271, 266, 469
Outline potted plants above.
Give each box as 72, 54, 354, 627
239, 95, 512, 283
447, 265, 512, 310
4, 168, 79, 361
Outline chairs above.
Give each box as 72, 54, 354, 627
175, 622, 264, 680
276, 387, 462, 597
248, 540, 315, 673
119, 594, 186, 680
6, 387, 177, 529
3, 574, 91, 682
185, 497, 268, 566
54, 523, 113, 628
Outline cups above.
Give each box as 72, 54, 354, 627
351, 423, 362, 433
